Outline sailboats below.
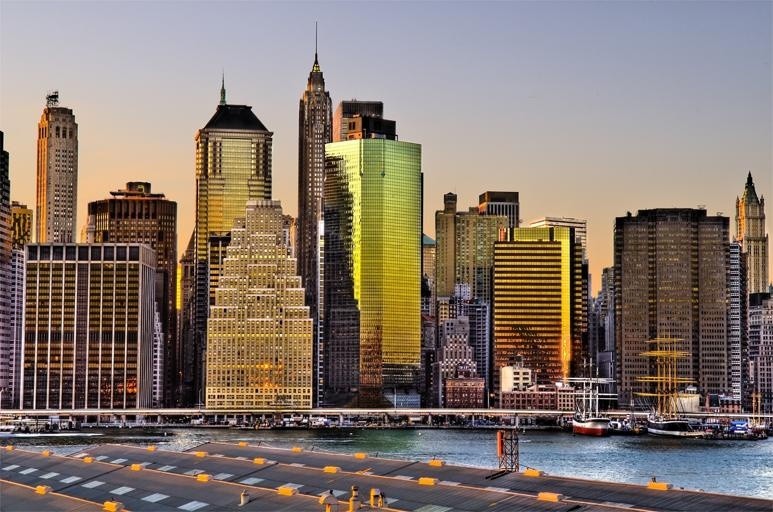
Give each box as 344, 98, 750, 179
630, 326, 706, 439
560, 358, 619, 436
